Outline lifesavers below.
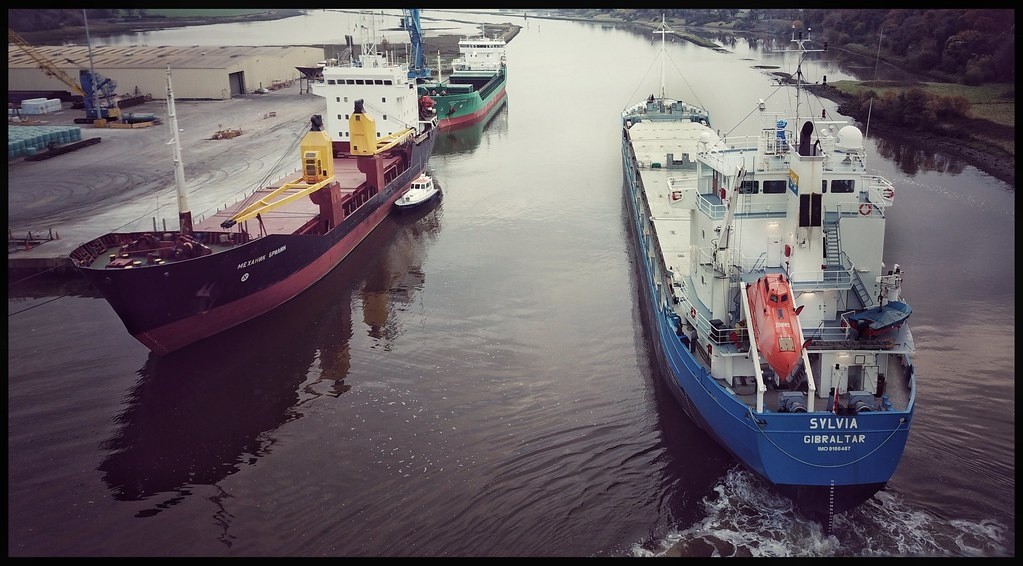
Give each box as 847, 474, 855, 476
691, 307, 696, 318
672, 190, 682, 200
859, 204, 871, 216
882, 189, 894, 199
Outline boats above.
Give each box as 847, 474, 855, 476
415, 31, 509, 142
393, 166, 440, 215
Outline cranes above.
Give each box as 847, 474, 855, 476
7, 29, 116, 109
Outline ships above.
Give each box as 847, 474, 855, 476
70, 23, 440, 367
622, 13, 919, 535
